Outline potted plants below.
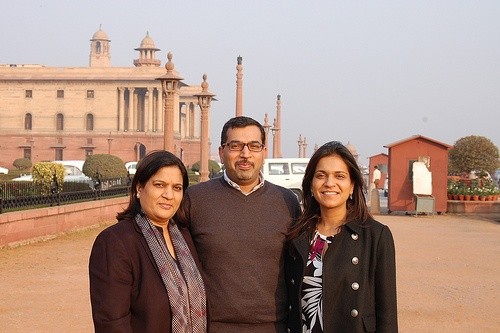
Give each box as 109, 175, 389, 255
448, 194, 498, 201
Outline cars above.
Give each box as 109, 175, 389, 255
124, 161, 140, 175
261, 158, 315, 204
11, 160, 94, 182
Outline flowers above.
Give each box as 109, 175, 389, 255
448, 184, 500, 194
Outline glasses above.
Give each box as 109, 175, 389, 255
223, 141, 265, 151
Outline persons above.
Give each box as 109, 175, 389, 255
372, 165, 382, 188
283, 139, 400, 333
89, 148, 209, 333
174, 115, 304, 333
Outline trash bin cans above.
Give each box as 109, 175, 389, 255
414, 194, 435, 216
